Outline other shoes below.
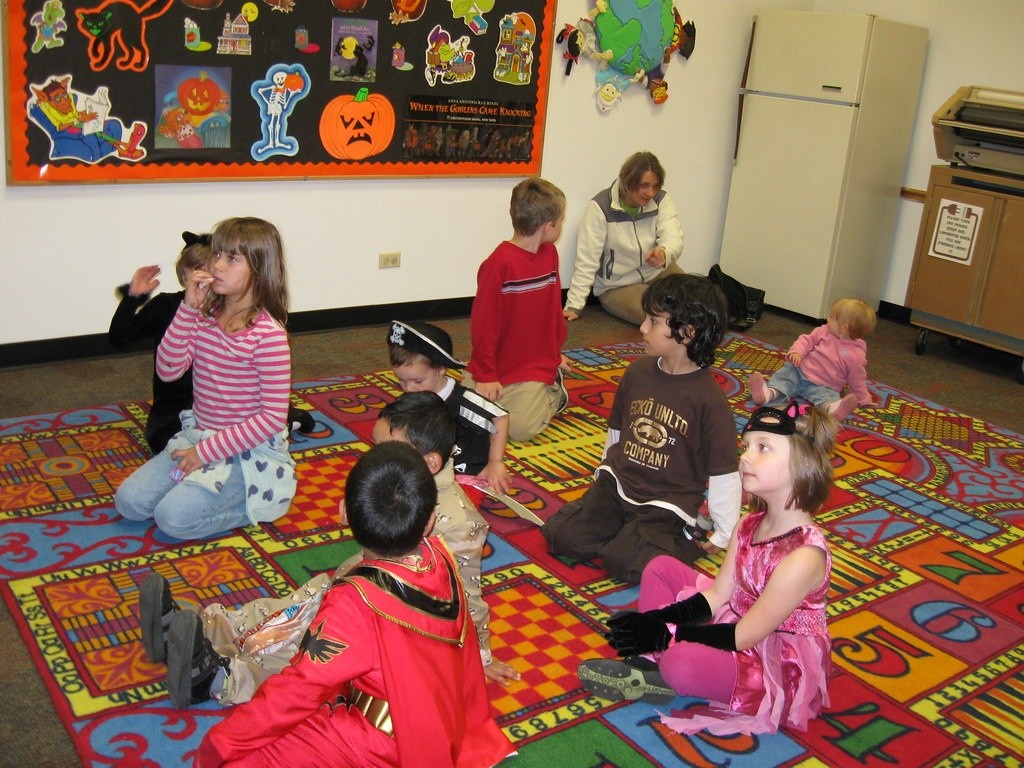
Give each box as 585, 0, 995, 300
481, 540, 495, 558
828, 394, 857, 423
577, 657, 676, 705
288, 404, 315, 433
749, 373, 770, 405
684, 513, 713, 544
555, 367, 569, 413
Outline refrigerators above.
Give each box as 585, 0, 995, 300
720, 8, 930, 323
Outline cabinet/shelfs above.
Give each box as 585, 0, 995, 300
905, 166, 1024, 384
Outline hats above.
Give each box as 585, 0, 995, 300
386, 319, 468, 369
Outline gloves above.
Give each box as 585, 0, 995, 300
613, 620, 737, 657
604, 593, 712, 648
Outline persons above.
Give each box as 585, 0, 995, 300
458, 177, 572, 442
113, 216, 297, 538
106, 233, 316, 453
602, 399, 842, 737
193, 439, 515, 768
749, 298, 878, 420
541, 272, 743, 581
138, 389, 520, 708
562, 150, 684, 326
383, 319, 513, 508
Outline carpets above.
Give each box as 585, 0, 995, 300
0, 332, 1024, 768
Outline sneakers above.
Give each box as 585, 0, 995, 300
140, 572, 184, 664
167, 610, 231, 708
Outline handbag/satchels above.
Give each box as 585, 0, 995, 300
709, 264, 765, 331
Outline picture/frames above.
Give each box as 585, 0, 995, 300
1, 0, 557, 187
